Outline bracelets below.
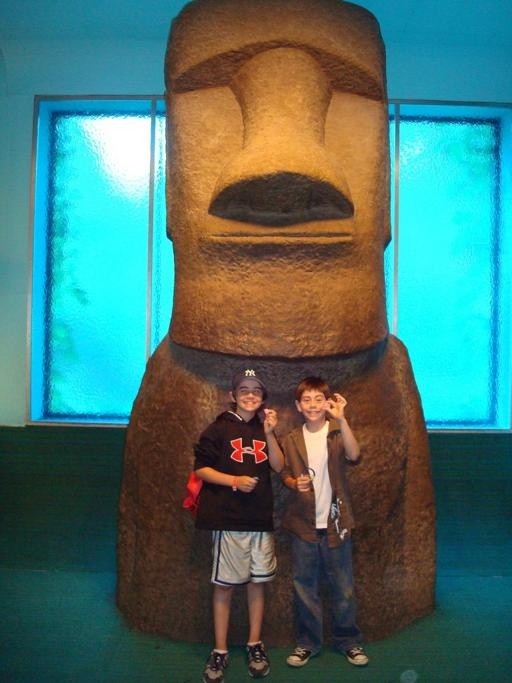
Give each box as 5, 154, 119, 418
231, 475, 240, 491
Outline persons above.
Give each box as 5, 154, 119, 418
191, 366, 286, 683
278, 374, 370, 667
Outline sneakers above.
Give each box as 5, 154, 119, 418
245, 641, 271, 679
286, 642, 322, 666
202, 649, 231, 683
336, 641, 368, 666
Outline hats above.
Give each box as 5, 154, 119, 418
232, 368, 266, 392
